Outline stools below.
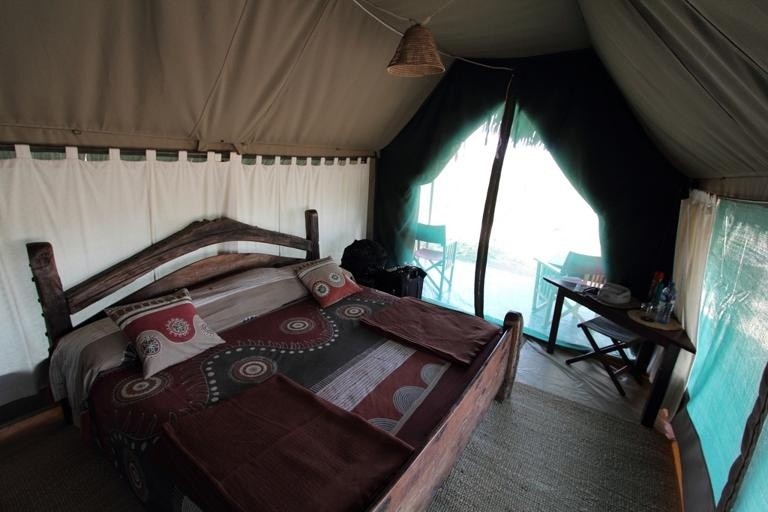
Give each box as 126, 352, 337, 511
565, 317, 642, 396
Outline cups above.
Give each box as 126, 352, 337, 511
641, 303, 655, 321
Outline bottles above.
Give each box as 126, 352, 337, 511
648, 272, 665, 311
656, 282, 678, 323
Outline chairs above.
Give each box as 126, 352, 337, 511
414, 222, 458, 299
532, 250, 606, 327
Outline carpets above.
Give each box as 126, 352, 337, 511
1, 381, 685, 512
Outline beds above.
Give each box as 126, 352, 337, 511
24, 208, 525, 511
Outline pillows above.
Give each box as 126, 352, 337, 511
293, 255, 358, 308
104, 290, 227, 379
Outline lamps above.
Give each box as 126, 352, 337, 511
388, 21, 448, 80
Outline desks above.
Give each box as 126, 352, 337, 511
541, 275, 692, 427
